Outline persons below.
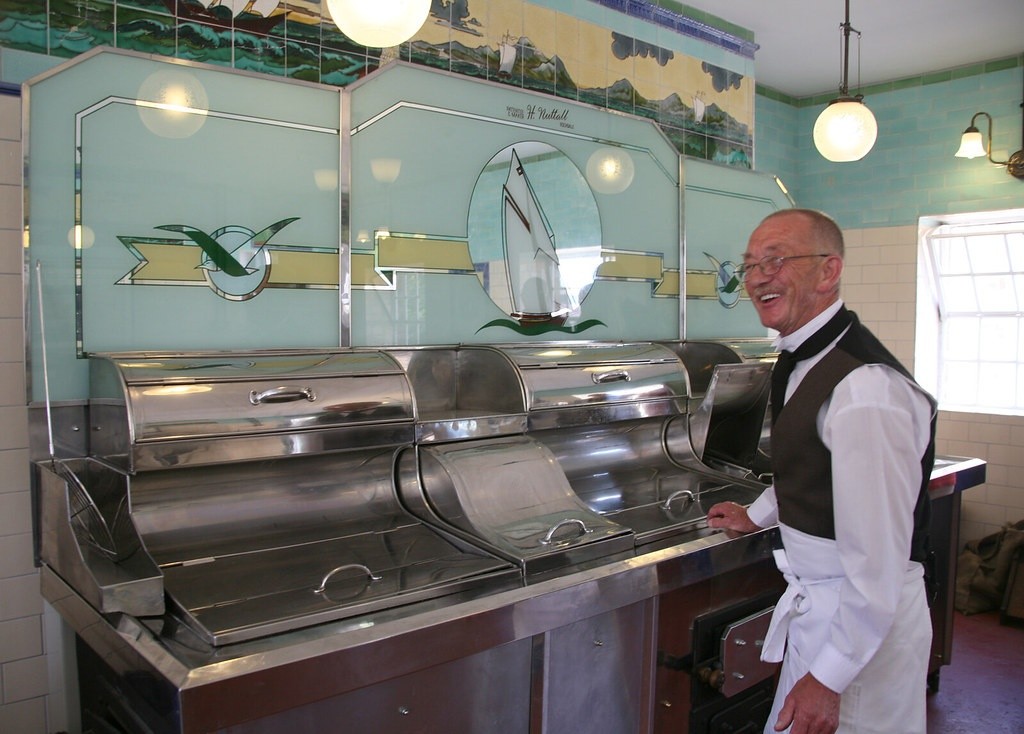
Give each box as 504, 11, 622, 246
707, 207, 935, 734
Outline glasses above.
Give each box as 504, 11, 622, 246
733, 254, 828, 282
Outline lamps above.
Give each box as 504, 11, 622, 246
68, 226, 96, 249
326, 0, 432, 48
356, 227, 371, 244
954, 112, 1024, 180
313, 167, 338, 192
134, 64, 209, 139
586, 146, 634, 197
375, 226, 392, 242
813, 0, 878, 163
372, 155, 403, 185
